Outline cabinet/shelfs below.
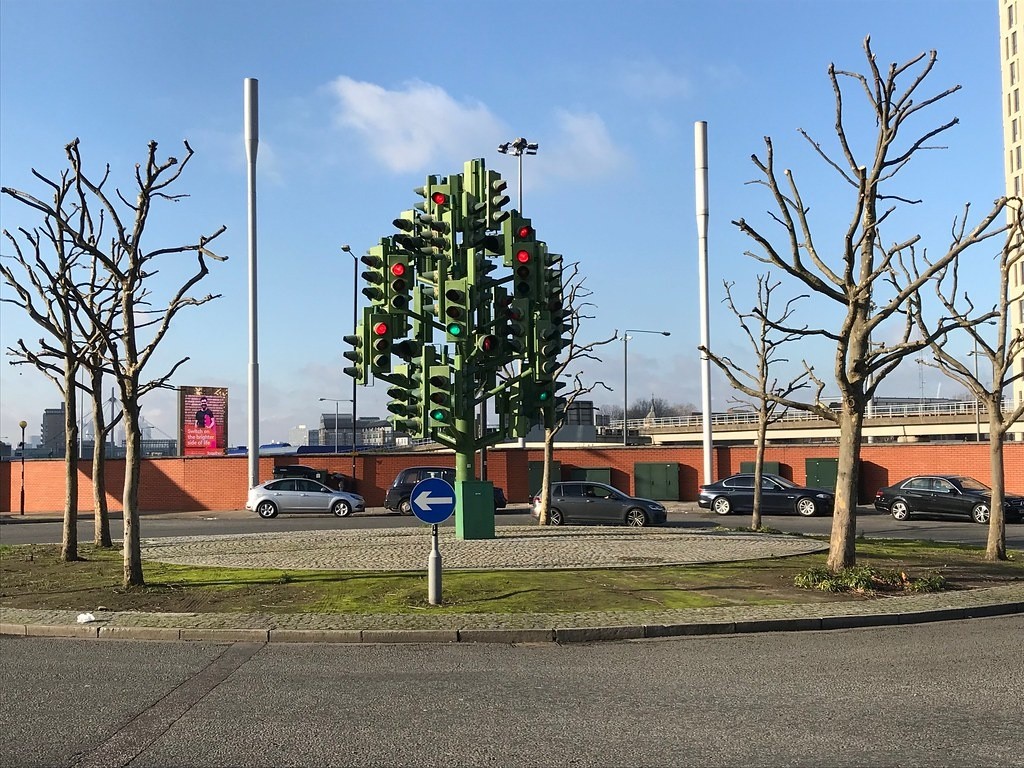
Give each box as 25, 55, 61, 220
804, 457, 863, 502
528, 460, 611, 497
740, 461, 780, 475
633, 462, 679, 501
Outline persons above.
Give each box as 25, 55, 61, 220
195, 396, 215, 429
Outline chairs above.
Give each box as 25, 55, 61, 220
588, 486, 595, 497
935, 481, 941, 491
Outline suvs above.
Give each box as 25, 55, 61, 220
382, 466, 508, 516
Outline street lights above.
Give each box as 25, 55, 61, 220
339, 243, 358, 488
973, 321, 998, 443
18, 421, 27, 516
623, 329, 671, 449
497, 137, 538, 215
317, 397, 353, 454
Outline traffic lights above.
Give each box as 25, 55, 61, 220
367, 312, 393, 378
387, 254, 411, 313
359, 244, 385, 306
388, 157, 576, 437
343, 325, 364, 385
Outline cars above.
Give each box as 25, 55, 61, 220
697, 473, 835, 517
245, 477, 366, 518
530, 481, 668, 528
874, 474, 1024, 525
